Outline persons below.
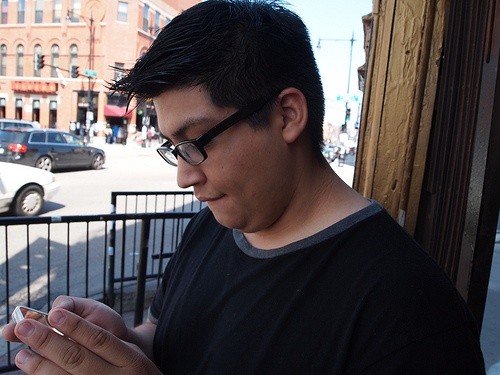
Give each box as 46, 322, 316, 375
68, 120, 159, 147
2, 0, 487, 375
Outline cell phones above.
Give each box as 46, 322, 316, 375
9, 306, 76, 343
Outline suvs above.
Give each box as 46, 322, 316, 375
0, 118, 42, 129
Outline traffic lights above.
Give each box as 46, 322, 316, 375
36, 52, 45, 70
71, 65, 80, 78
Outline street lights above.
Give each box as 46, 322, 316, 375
316, 34, 356, 133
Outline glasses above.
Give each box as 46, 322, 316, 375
156, 92, 280, 168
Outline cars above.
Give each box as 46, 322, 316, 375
0, 159, 62, 215
0, 127, 106, 172
